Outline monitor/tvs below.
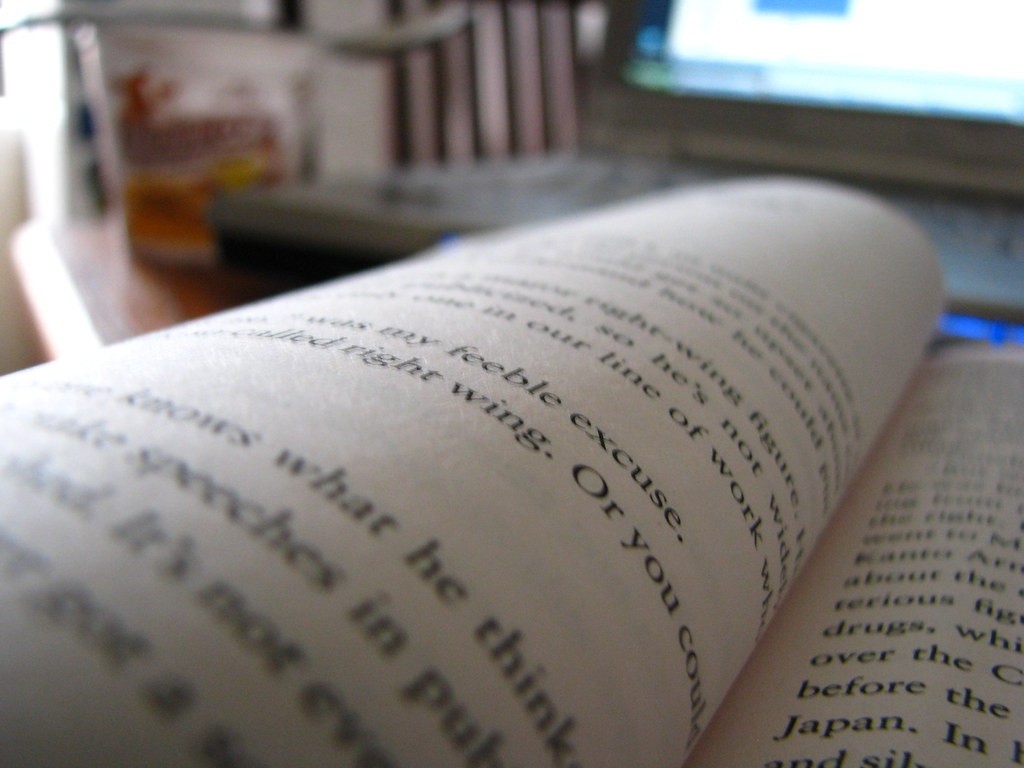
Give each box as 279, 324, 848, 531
583, 0, 1024, 204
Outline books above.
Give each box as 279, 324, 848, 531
0, 169, 1024, 768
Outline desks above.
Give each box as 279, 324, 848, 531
209, 154, 1024, 302
